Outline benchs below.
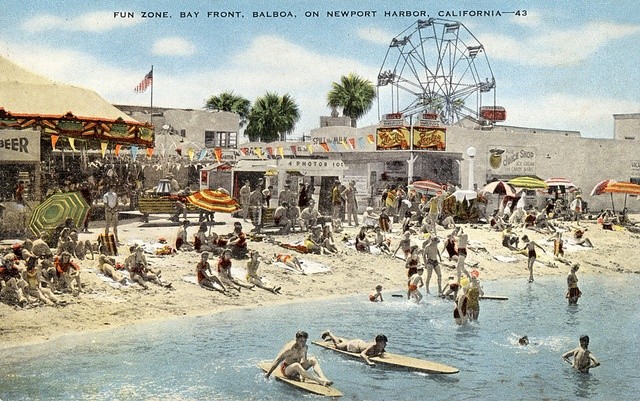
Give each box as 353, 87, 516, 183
137, 196, 217, 225
248, 206, 312, 233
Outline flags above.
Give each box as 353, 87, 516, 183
134, 71, 152, 94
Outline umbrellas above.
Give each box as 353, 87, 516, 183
507, 176, 548, 193
478, 180, 516, 209
590, 179, 621, 200
537, 178, 581, 199
29, 190, 91, 238
603, 179, 640, 199
186, 189, 243, 235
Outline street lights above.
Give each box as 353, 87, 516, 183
467, 147, 476, 191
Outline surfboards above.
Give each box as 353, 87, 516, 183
444, 291, 507, 300
260, 362, 343, 398
312, 337, 459, 374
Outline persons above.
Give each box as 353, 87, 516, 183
176, 220, 195, 251
197, 253, 229, 295
407, 268, 424, 302
265, 331, 333, 387
501, 224, 520, 251
422, 237, 444, 293
272, 252, 304, 272
103, 153, 120, 194
400, 178, 478, 234
11, 244, 23, 261
249, 179, 399, 253
57, 228, 76, 258
321, 329, 388, 366
422, 234, 455, 268
519, 336, 529, 346
464, 270, 484, 320
99, 246, 127, 284
21, 239, 39, 261
547, 231, 572, 265
225, 225, 247, 258
0, 155, 100, 205
369, 285, 383, 302
562, 335, 600, 373
103, 184, 120, 242
440, 276, 461, 300
454, 277, 471, 323
405, 245, 421, 299
195, 223, 215, 252
247, 251, 281, 293
240, 180, 251, 222
517, 235, 546, 282
207, 233, 228, 256
21, 256, 67, 306
39, 253, 65, 294
70, 232, 94, 259
218, 250, 255, 291
0, 253, 38, 303
440, 234, 478, 267
567, 264, 582, 305
574, 230, 593, 247
504, 195, 640, 235
56, 252, 86, 294
393, 231, 411, 259
120, 155, 146, 211
125, 246, 172, 289
32, 235, 57, 259
130, 246, 161, 278
228, 222, 241, 237
165, 172, 230, 222
